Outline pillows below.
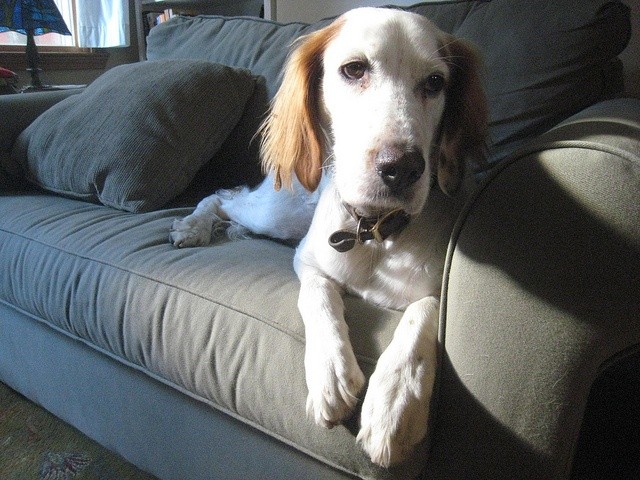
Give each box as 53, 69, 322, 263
1, 58, 259, 214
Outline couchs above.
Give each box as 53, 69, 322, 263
0, 0, 640, 480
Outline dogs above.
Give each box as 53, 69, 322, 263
167, 5, 497, 470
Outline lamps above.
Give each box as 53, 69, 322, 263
0, 1, 72, 88
